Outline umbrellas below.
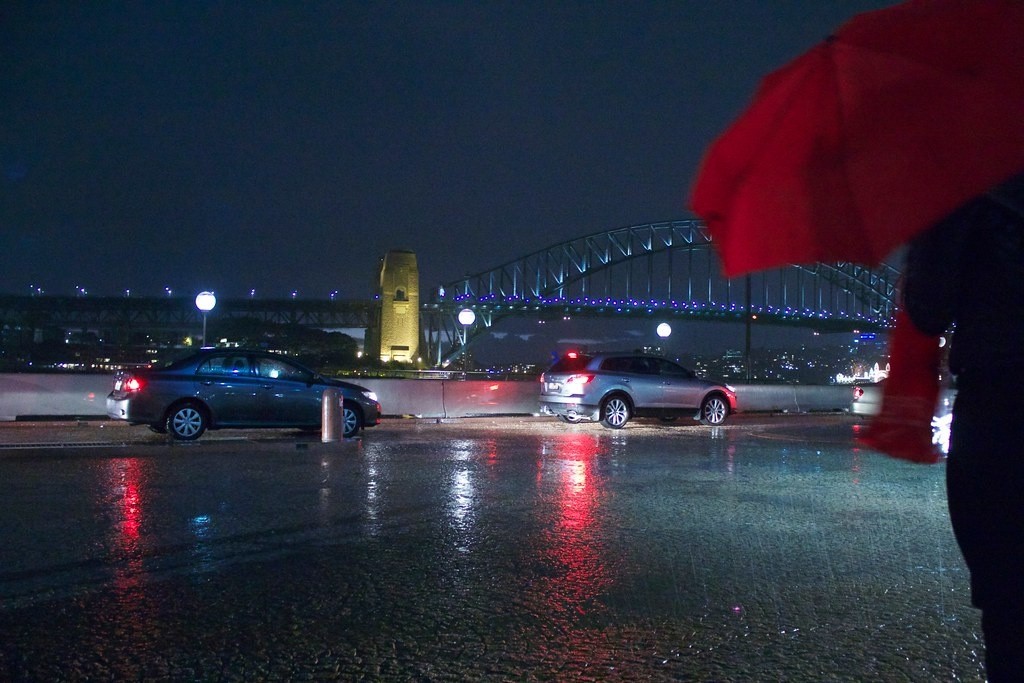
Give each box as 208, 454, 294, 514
688, 0, 1024, 277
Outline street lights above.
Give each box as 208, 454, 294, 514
656, 322, 672, 357
195, 291, 216, 347
458, 309, 476, 380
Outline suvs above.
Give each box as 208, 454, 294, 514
539, 351, 739, 427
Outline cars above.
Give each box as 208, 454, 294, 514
851, 369, 956, 418
106, 347, 381, 440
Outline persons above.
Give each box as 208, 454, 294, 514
903, 172, 1024, 683
